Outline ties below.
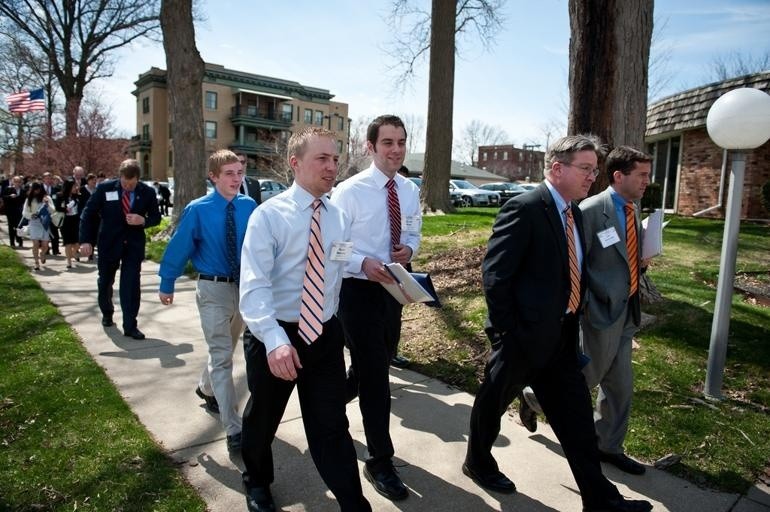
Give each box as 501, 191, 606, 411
298, 199, 324, 345
224, 202, 238, 278
384, 177, 404, 265
120, 190, 129, 214
624, 201, 640, 298
239, 181, 245, 195
46, 185, 50, 196
561, 204, 580, 314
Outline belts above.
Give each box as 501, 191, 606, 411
197, 273, 239, 283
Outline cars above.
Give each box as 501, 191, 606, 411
207, 180, 214, 196
449, 179, 501, 207
518, 183, 540, 191
407, 178, 461, 207
168, 184, 174, 204
257, 179, 288, 202
478, 182, 529, 205
143, 181, 170, 204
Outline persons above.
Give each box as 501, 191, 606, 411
0, 159, 171, 340
157, 149, 259, 457
331, 115, 424, 499
517, 145, 653, 475
241, 127, 374, 512
461, 130, 652, 510
230, 148, 262, 206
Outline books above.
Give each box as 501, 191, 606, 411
377, 262, 435, 306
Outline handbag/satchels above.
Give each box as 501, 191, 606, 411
50, 211, 65, 227
16, 215, 31, 239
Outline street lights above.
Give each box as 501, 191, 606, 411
704, 87, 770, 396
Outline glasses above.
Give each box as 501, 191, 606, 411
554, 160, 601, 178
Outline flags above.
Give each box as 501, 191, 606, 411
6, 88, 45, 114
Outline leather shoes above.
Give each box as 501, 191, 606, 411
461, 459, 516, 496
124, 327, 145, 341
244, 481, 273, 511
580, 494, 653, 511
194, 386, 219, 413
363, 458, 410, 502
224, 431, 243, 451
102, 316, 113, 326
595, 449, 648, 476
515, 389, 538, 431
